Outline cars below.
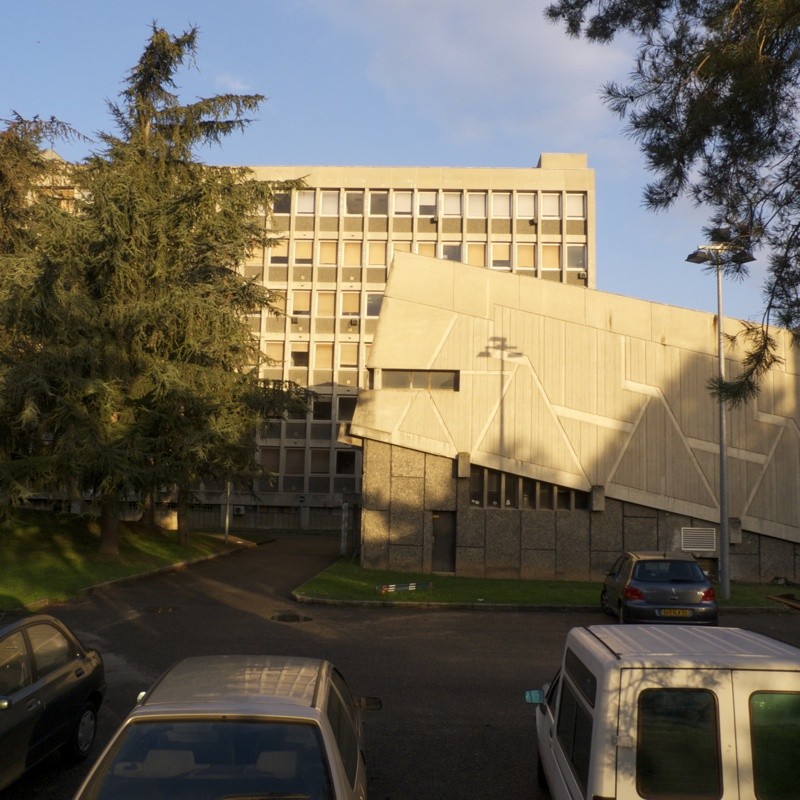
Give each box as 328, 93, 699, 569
601, 550, 719, 627
0, 608, 107, 791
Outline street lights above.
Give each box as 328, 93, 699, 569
684, 242, 756, 604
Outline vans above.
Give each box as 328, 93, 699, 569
68, 652, 382, 800
526, 625, 800, 800
